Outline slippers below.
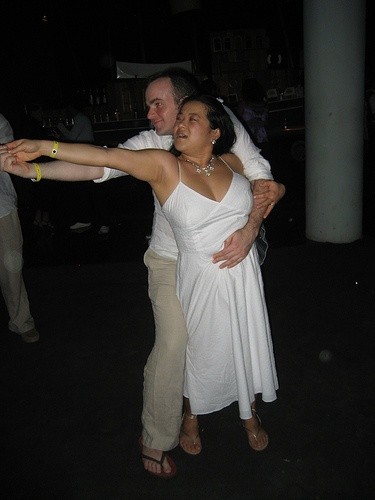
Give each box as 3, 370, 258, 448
141, 439, 176, 477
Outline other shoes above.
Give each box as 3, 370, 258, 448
70, 221, 90, 230
21, 329, 40, 343
99, 225, 109, 234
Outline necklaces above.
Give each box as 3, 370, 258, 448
185, 154, 216, 176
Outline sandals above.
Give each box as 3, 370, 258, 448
180, 414, 202, 456
244, 409, 269, 452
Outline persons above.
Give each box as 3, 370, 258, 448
0, 70, 288, 477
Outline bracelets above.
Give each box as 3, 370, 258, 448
31, 163, 42, 182
50, 141, 58, 158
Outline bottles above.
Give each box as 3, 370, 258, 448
40, 79, 147, 131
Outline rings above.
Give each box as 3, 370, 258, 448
268, 202, 275, 208
